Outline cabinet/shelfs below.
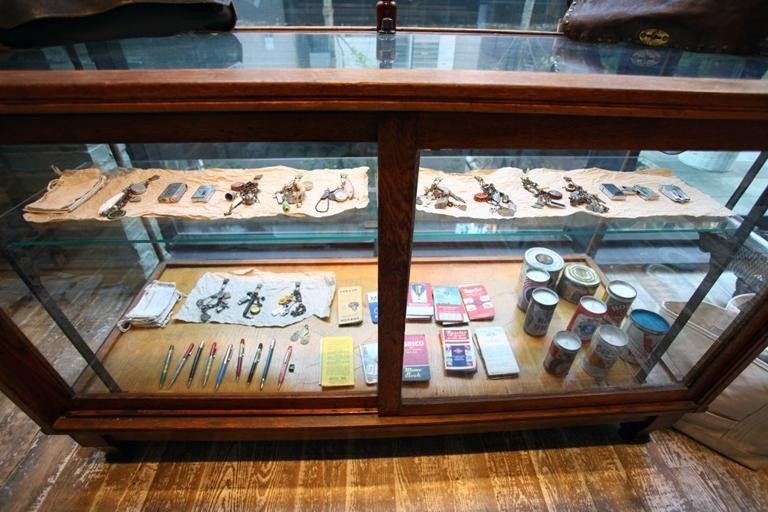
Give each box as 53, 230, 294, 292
1, 0, 768, 452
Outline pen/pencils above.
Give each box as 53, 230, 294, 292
159, 336, 292, 392
